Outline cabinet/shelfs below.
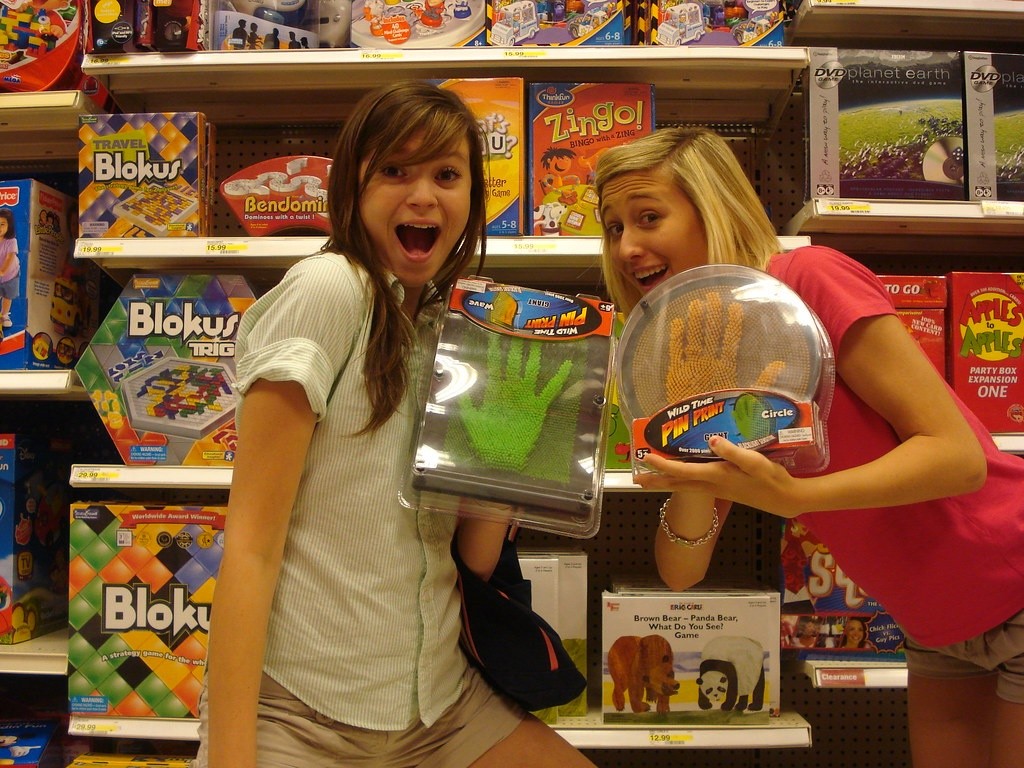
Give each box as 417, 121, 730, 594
0, 90, 86, 675
69, 45, 830, 748
786, 0, 1024, 688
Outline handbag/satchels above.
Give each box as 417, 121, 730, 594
450, 519, 590, 713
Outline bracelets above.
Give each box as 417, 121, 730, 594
659, 498, 719, 549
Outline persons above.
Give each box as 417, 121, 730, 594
840, 618, 866, 648
190, 78, 599, 768
790, 617, 817, 648
591, 125, 1024, 768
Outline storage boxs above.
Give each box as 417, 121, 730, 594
68, 0, 782, 720
0, 0, 89, 768
810, 46, 1024, 433
